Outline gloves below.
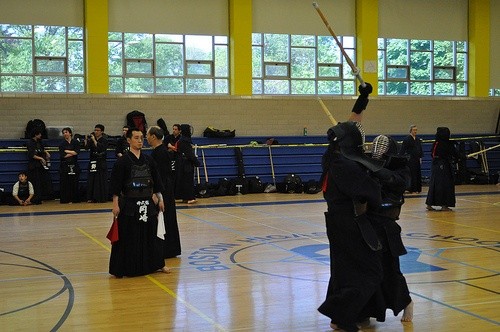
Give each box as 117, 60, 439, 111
369, 167, 393, 183
351, 82, 372, 114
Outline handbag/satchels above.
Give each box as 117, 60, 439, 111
194, 174, 321, 198
203, 126, 236, 138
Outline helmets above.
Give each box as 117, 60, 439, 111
329, 121, 365, 155
371, 134, 398, 162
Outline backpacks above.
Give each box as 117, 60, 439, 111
127, 110, 147, 137
25, 119, 46, 139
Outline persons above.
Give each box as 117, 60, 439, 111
59, 127, 80, 203
425, 126, 456, 212
83, 124, 109, 204
106, 126, 173, 278
370, 134, 415, 322
115, 126, 130, 154
146, 126, 182, 258
319, 80, 393, 332
28, 127, 51, 205
169, 124, 180, 146
399, 125, 424, 195
174, 125, 200, 204
156, 118, 171, 148
12, 172, 34, 205
